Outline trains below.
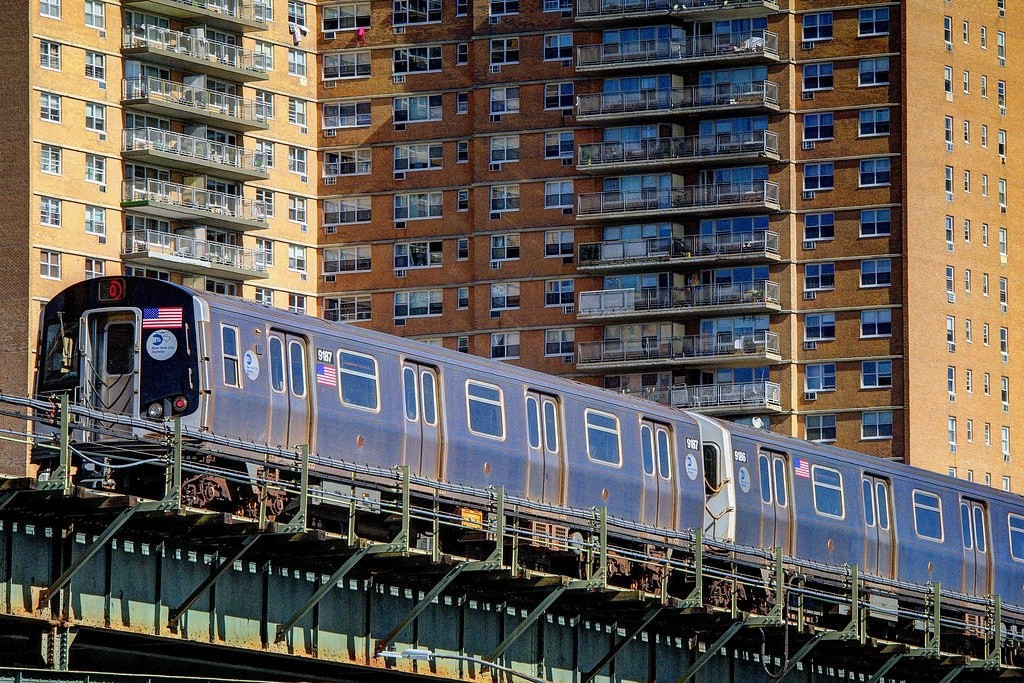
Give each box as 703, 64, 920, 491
29, 273, 1023, 670
